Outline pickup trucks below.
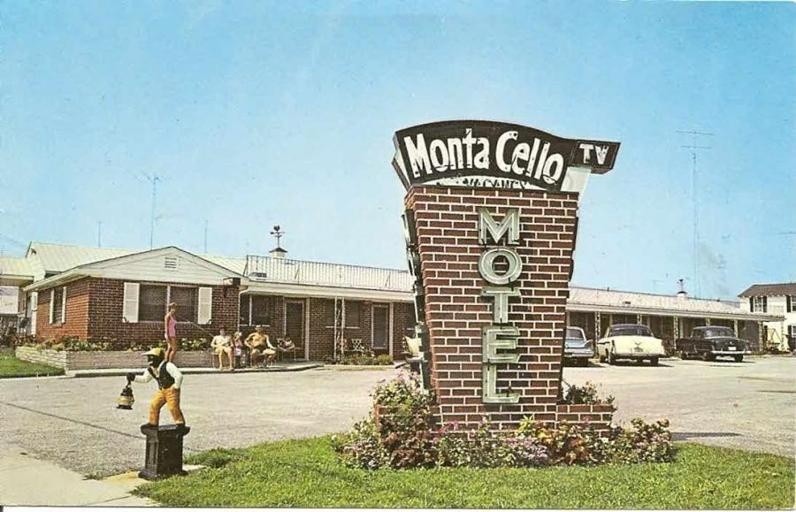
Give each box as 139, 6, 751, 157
676, 326, 753, 361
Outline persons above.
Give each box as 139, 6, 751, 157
230, 331, 246, 369
125, 344, 185, 433
210, 325, 235, 373
243, 322, 277, 368
162, 302, 179, 364
277, 333, 295, 354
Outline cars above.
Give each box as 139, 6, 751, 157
564, 326, 594, 367
595, 323, 667, 365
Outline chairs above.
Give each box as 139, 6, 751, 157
276, 338, 296, 361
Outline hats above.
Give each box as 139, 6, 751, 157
141, 347, 165, 358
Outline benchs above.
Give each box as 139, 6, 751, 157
207, 347, 276, 368
